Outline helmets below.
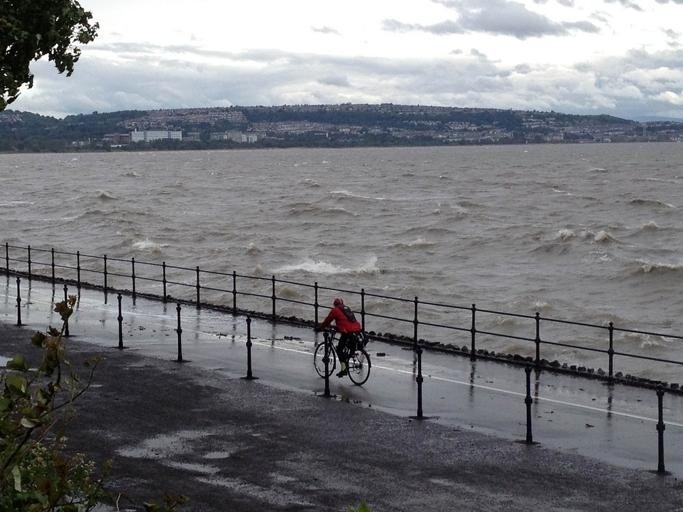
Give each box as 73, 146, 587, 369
333, 298, 343, 305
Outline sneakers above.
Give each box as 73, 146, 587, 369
336, 370, 347, 376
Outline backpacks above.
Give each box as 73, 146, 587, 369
343, 306, 356, 322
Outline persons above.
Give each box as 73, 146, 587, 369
315, 298, 360, 376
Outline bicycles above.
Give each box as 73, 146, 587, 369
311, 324, 370, 388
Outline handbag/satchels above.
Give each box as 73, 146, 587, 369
348, 329, 370, 350
